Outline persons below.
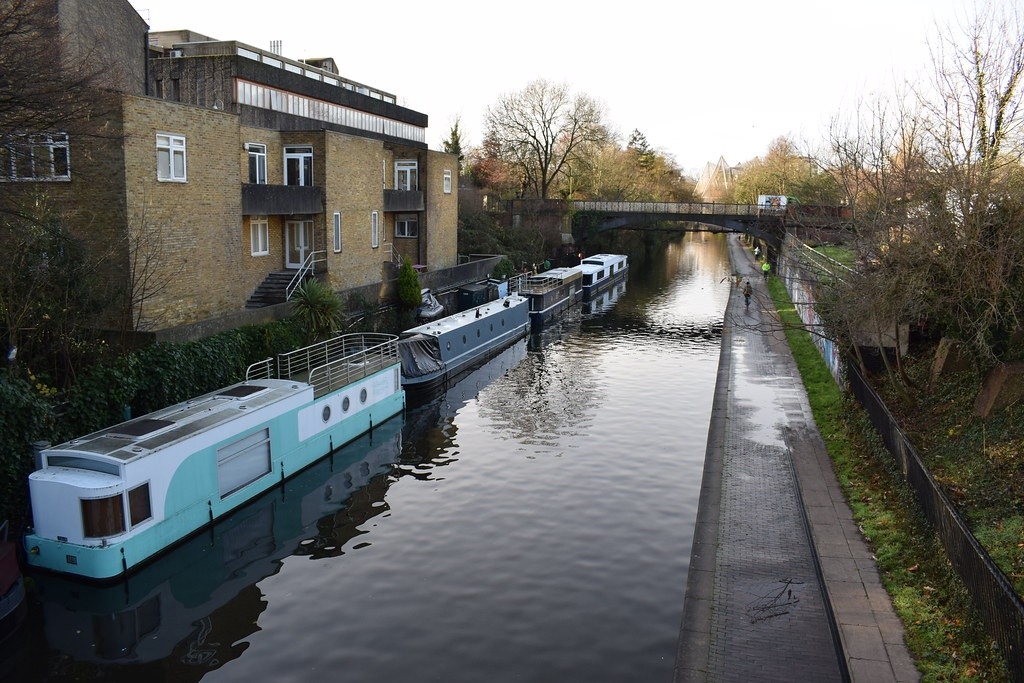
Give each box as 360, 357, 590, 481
762, 261, 770, 280
743, 282, 753, 305
754, 247, 760, 260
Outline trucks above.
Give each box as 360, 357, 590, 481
757, 195, 802, 217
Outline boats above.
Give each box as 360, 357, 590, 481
374, 274, 531, 386
23, 407, 407, 668
569, 251, 630, 298
391, 323, 533, 422
579, 279, 627, 320
22, 333, 408, 582
505, 267, 584, 321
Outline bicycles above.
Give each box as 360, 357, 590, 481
763, 270, 768, 280
743, 293, 751, 311
